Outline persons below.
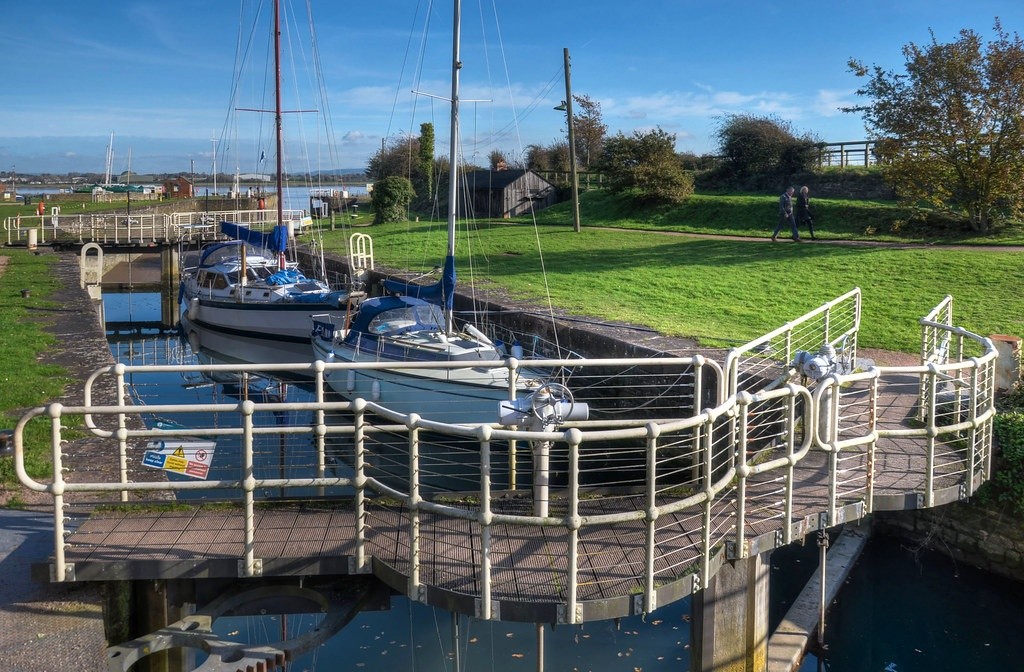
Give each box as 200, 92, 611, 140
772, 186, 801, 242
248, 187, 252, 197
795, 186, 818, 241
257, 186, 261, 197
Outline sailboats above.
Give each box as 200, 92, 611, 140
177, 1, 369, 337
310, 1, 585, 425
177, 309, 322, 497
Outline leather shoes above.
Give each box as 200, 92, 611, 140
771, 236, 776, 242
812, 236, 818, 241
792, 237, 802, 242
